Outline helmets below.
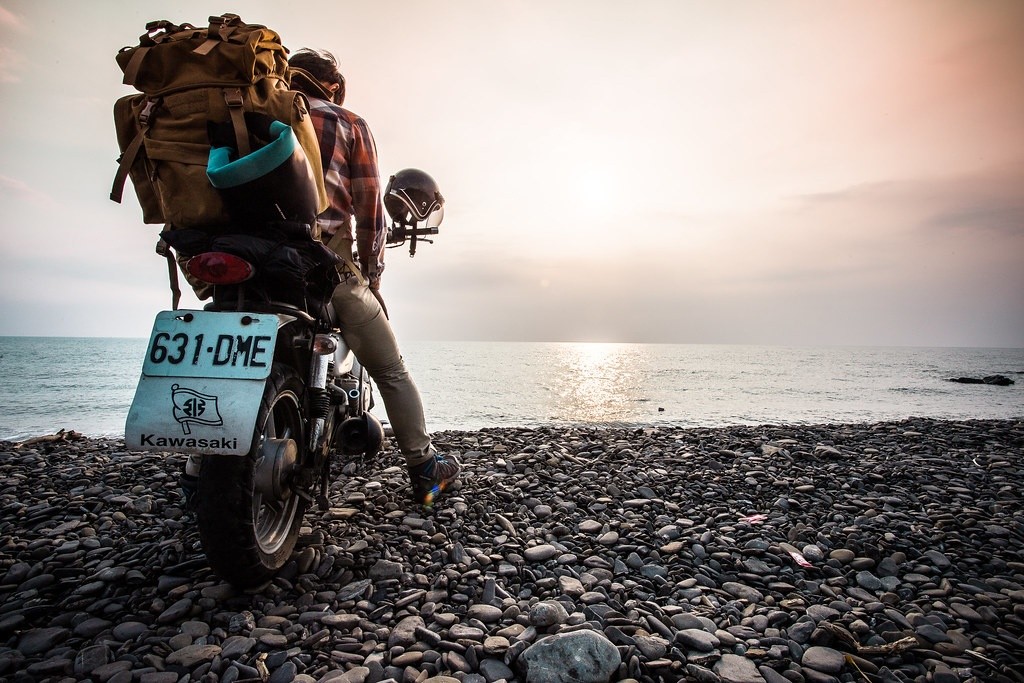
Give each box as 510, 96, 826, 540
383, 168, 446, 226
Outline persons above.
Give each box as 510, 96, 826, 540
181, 48, 461, 506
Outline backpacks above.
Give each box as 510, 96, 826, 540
113, 11, 345, 312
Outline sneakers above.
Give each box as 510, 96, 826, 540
407, 455, 461, 505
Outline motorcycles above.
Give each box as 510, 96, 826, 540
126, 215, 442, 589
